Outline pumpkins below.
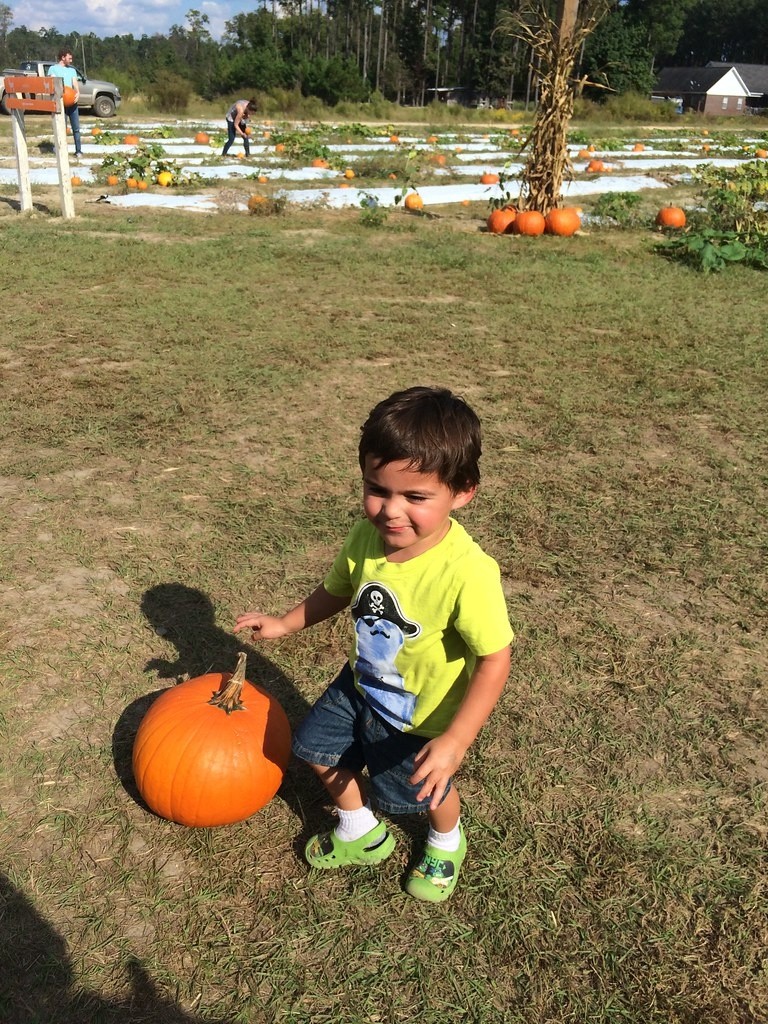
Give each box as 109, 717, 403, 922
132, 652, 292, 825
63, 86, 76, 106
65, 126, 767, 236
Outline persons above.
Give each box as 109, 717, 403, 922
221, 96, 259, 158
47, 48, 85, 159
231, 388, 517, 904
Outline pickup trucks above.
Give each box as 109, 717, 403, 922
0, 60, 122, 117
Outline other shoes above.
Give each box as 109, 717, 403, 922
76, 153, 82, 158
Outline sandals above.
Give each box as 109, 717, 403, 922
305, 820, 396, 870
405, 822, 467, 903
